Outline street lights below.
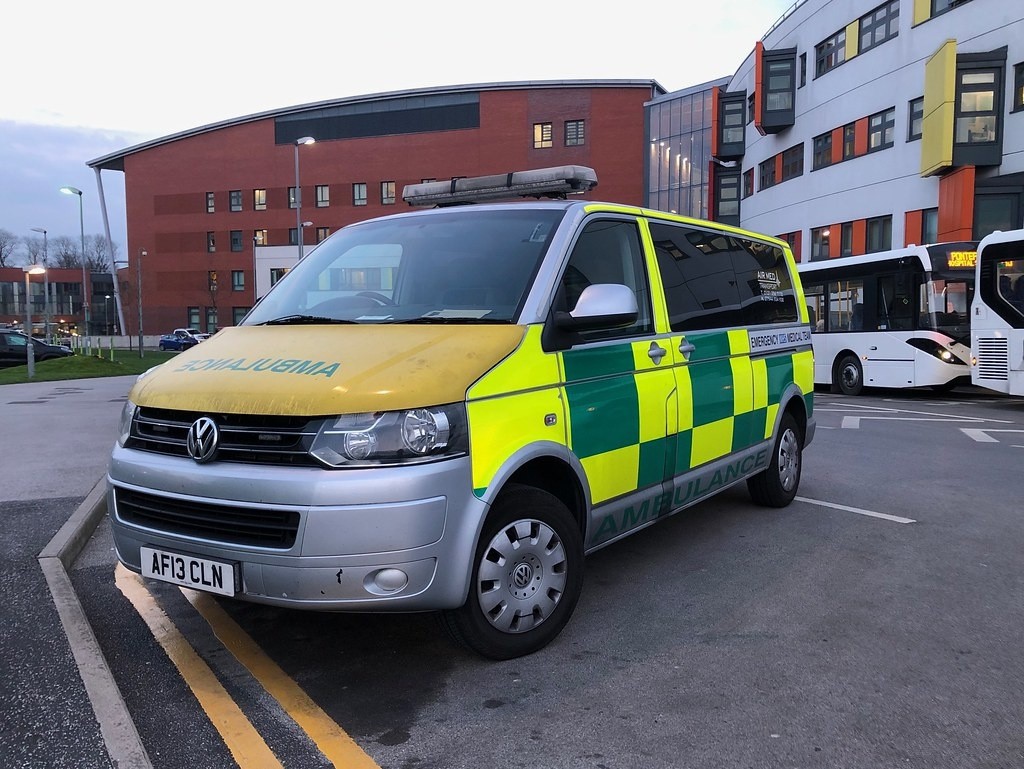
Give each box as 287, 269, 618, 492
252, 236, 264, 301
60, 187, 91, 356
293, 137, 315, 260
23, 227, 50, 379
137, 247, 148, 358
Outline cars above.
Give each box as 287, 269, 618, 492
0, 330, 75, 370
160, 328, 214, 351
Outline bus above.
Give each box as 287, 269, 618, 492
796, 230, 1024, 396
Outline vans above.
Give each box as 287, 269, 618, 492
105, 164, 817, 657
